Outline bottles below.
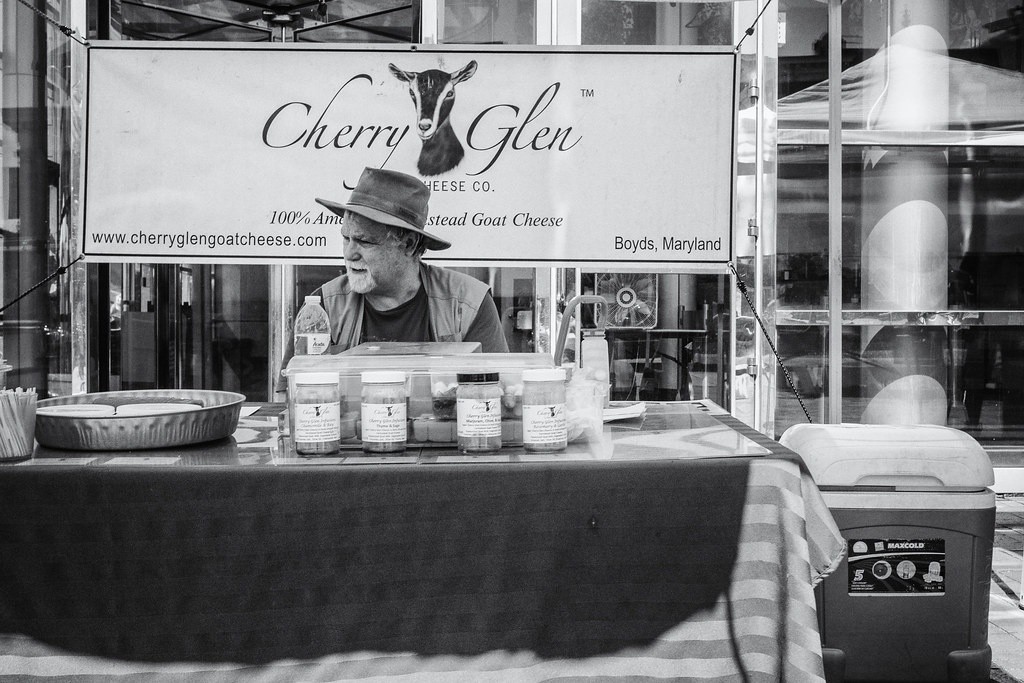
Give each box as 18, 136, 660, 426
360, 371, 408, 453
294, 296, 331, 355
522, 369, 567, 452
456, 372, 502, 452
294, 372, 341, 455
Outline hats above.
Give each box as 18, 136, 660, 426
314, 166, 451, 250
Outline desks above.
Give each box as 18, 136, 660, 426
0, 401, 850, 683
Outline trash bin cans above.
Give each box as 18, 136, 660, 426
778, 423, 996, 683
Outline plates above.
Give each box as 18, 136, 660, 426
116, 402, 201, 417
37, 404, 115, 417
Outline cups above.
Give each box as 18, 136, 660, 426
0, 390, 39, 461
567, 394, 609, 444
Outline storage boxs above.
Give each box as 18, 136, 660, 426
281, 355, 557, 445
338, 340, 483, 354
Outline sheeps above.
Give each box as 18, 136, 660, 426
388, 59, 478, 176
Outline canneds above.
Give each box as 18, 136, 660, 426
295, 373, 341, 455
360, 372, 406, 452
523, 369, 568, 453
455, 372, 502, 453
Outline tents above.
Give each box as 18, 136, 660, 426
738, 48, 1024, 159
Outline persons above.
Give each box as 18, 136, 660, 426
276, 168, 510, 403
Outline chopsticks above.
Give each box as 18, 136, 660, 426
0, 387, 36, 458
